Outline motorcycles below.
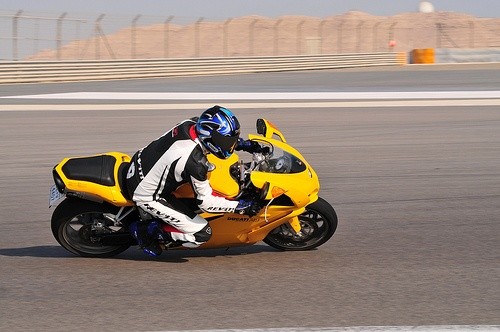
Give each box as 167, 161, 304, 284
48, 118, 339, 259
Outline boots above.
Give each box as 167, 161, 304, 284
129, 218, 163, 259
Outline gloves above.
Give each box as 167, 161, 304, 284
236, 200, 260, 218
236, 136, 254, 152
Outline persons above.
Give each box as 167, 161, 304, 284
123, 105, 264, 257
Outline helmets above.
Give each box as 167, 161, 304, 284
196, 105, 240, 159
